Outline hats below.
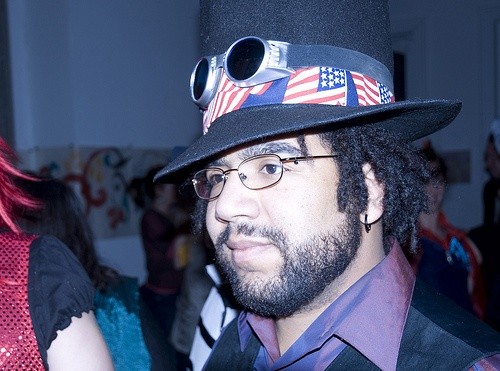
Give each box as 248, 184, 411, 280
152, 1, 464, 183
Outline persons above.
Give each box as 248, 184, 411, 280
396, 142, 482, 314
154, 0, 500, 371
0, 133, 150, 371
124, 162, 192, 357
464, 119, 500, 335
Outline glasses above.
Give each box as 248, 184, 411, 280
189, 36, 295, 111
192, 153, 341, 200
